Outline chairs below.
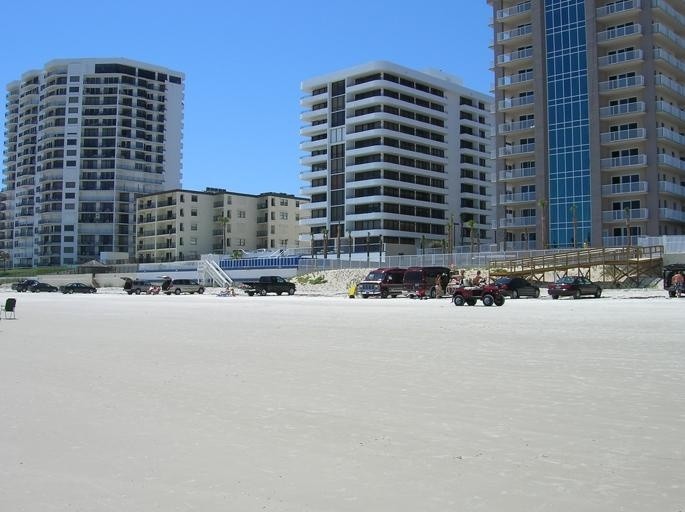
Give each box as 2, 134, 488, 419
0, 297, 17, 319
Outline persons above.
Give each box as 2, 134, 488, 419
147, 284, 154, 293
151, 286, 160, 294
460, 266, 466, 283
435, 273, 444, 299
218, 286, 228, 295
672, 270, 684, 298
473, 270, 483, 286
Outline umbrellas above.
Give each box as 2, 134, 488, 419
80, 260, 108, 277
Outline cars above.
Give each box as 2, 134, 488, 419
59, 283, 97, 294
494, 277, 541, 298
664, 264, 685, 297
12, 280, 58, 293
548, 276, 602, 299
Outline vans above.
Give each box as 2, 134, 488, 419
358, 267, 452, 298
119, 275, 205, 295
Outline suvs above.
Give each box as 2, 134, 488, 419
453, 279, 505, 305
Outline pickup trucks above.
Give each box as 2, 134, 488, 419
240, 276, 296, 296
